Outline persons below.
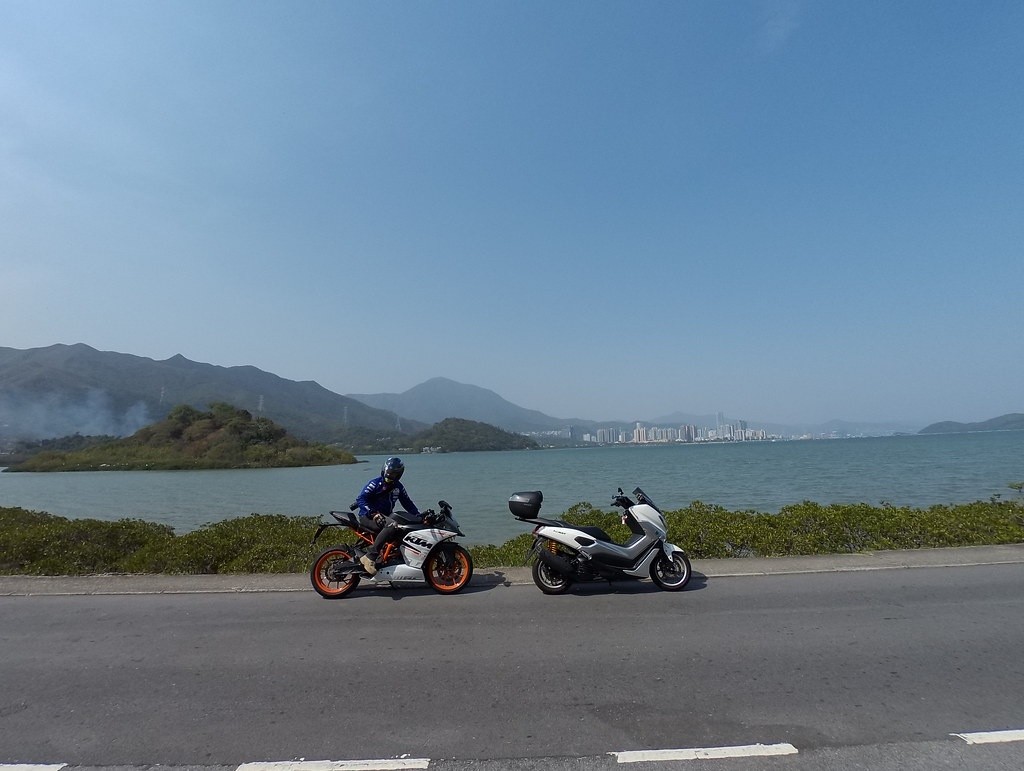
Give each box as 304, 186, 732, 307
355, 458, 421, 575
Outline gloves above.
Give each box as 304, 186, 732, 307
373, 512, 387, 529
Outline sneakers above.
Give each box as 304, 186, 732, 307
359, 555, 377, 576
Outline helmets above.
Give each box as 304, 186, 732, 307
383, 458, 404, 485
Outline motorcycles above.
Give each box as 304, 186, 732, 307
310, 500, 473, 600
508, 486, 692, 595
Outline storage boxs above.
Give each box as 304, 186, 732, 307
508, 491, 544, 519
